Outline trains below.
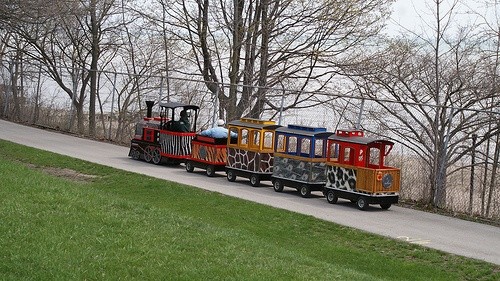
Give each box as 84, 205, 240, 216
129, 99, 400, 210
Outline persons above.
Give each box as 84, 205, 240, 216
201, 119, 239, 137
179, 110, 191, 132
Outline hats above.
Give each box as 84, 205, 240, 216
217, 119, 224, 125
